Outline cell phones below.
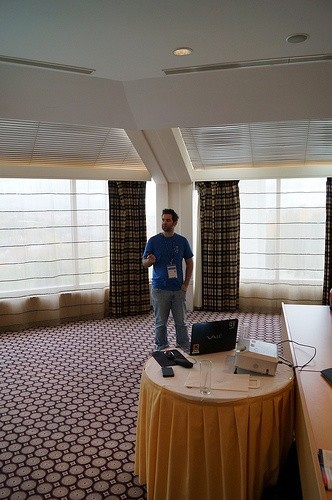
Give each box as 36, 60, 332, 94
161, 367, 174, 376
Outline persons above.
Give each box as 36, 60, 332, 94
143, 209, 194, 349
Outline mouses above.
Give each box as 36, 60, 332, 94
164, 350, 174, 359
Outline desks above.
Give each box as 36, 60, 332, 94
134, 344, 295, 500
280, 301, 332, 500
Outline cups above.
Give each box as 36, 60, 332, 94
199, 359, 212, 395
240, 324, 248, 339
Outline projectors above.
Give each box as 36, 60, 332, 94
234, 337, 277, 377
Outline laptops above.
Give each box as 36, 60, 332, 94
176, 318, 238, 355
